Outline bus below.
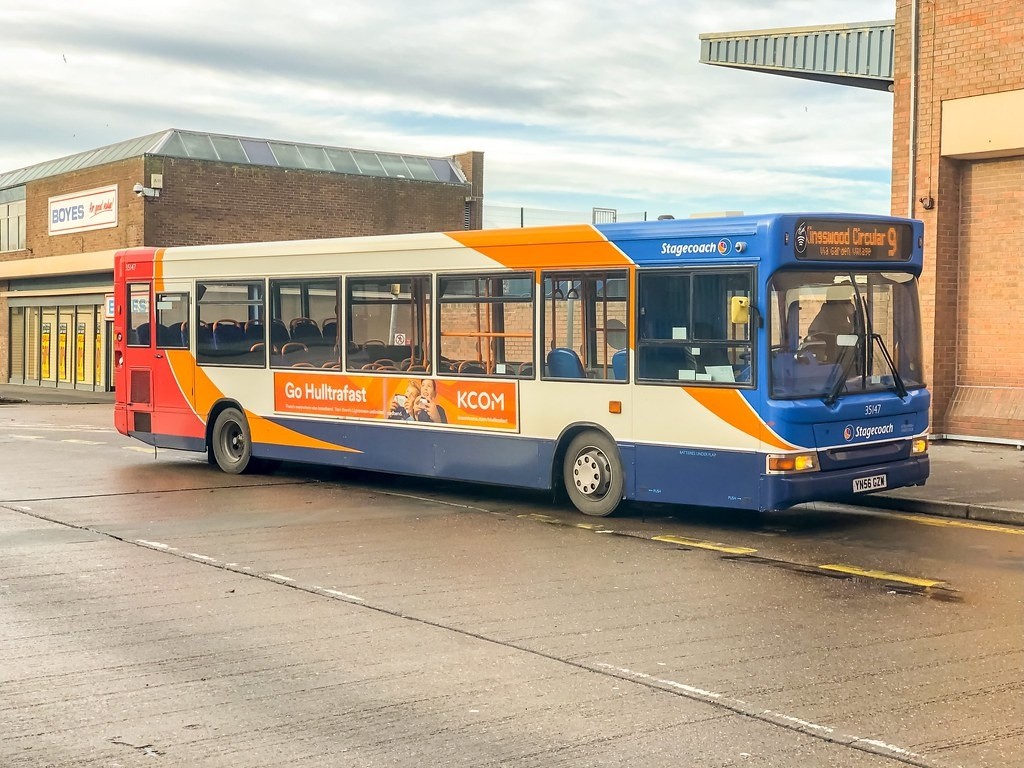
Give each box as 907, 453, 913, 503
113, 213, 929, 518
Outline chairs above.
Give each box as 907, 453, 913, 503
135, 317, 626, 381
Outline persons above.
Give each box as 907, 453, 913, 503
813, 283, 867, 368
389, 379, 447, 425
